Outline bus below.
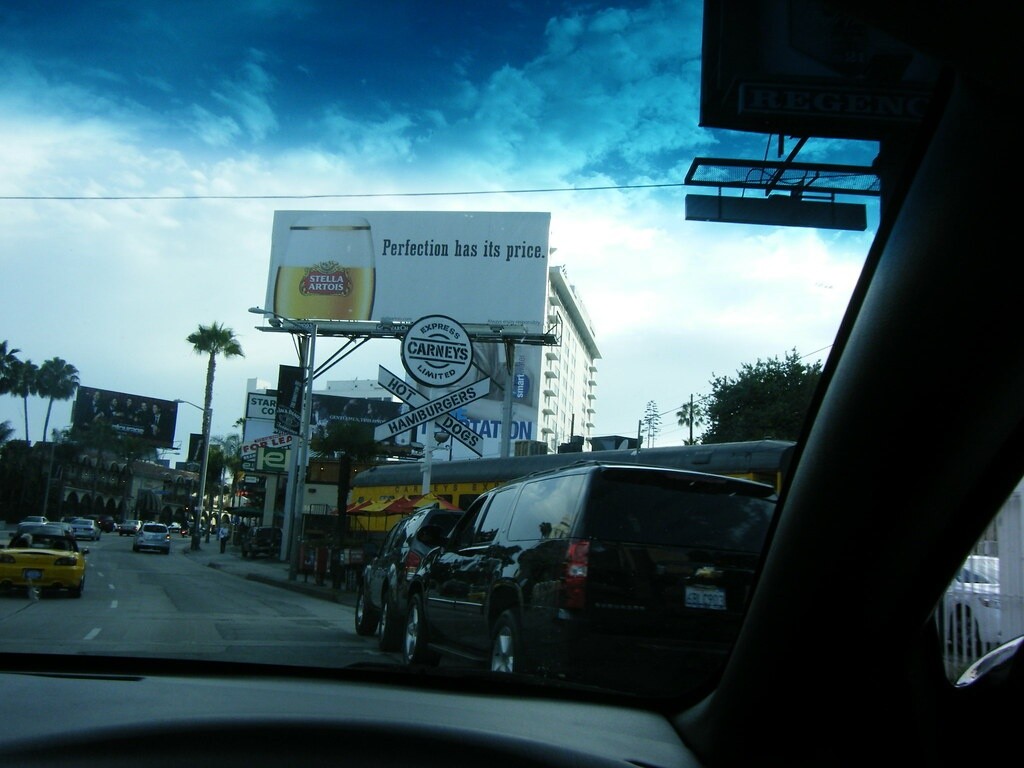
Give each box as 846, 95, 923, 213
345, 438, 800, 597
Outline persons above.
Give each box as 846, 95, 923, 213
181, 517, 189, 533
219, 514, 231, 554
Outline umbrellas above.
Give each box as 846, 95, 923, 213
330, 493, 461, 531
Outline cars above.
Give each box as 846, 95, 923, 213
117, 520, 143, 537
86, 513, 114, 535
45, 519, 77, 543
167, 521, 183, 533
20, 515, 50, 529
0, 523, 90, 597
68, 518, 103, 542
134, 521, 170, 553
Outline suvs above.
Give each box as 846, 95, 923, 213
356, 502, 468, 653
407, 452, 780, 711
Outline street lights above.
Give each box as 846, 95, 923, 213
173, 399, 213, 554
248, 307, 318, 585
